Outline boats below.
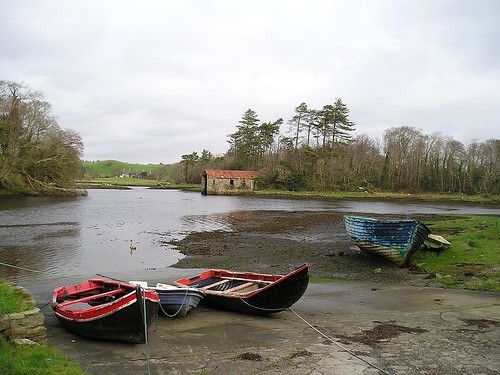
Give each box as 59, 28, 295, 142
173, 263, 310, 316
342, 214, 431, 269
128, 280, 206, 319
51, 278, 160, 344
